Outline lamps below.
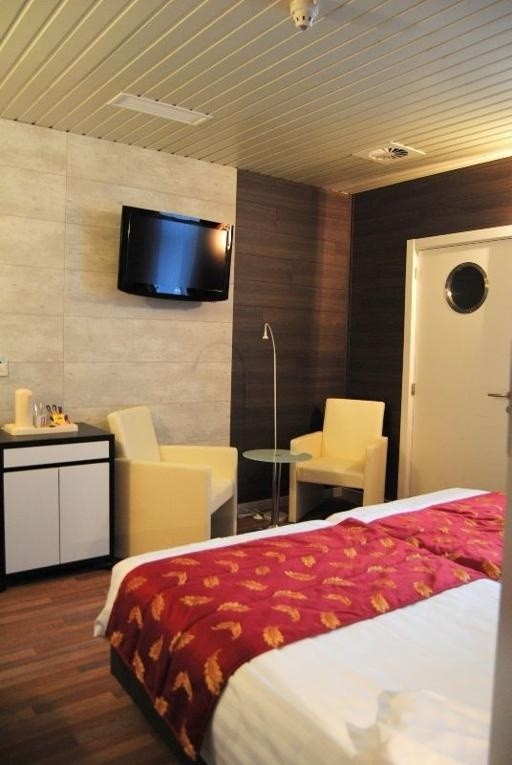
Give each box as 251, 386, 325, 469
260, 322, 279, 528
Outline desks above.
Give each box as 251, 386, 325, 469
240, 446, 312, 530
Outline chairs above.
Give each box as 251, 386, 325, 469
288, 398, 388, 523
106, 405, 239, 558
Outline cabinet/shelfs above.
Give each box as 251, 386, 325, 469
1, 421, 116, 587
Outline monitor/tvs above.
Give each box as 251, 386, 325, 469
116, 205, 234, 303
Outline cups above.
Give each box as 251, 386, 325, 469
33, 415, 47, 428
